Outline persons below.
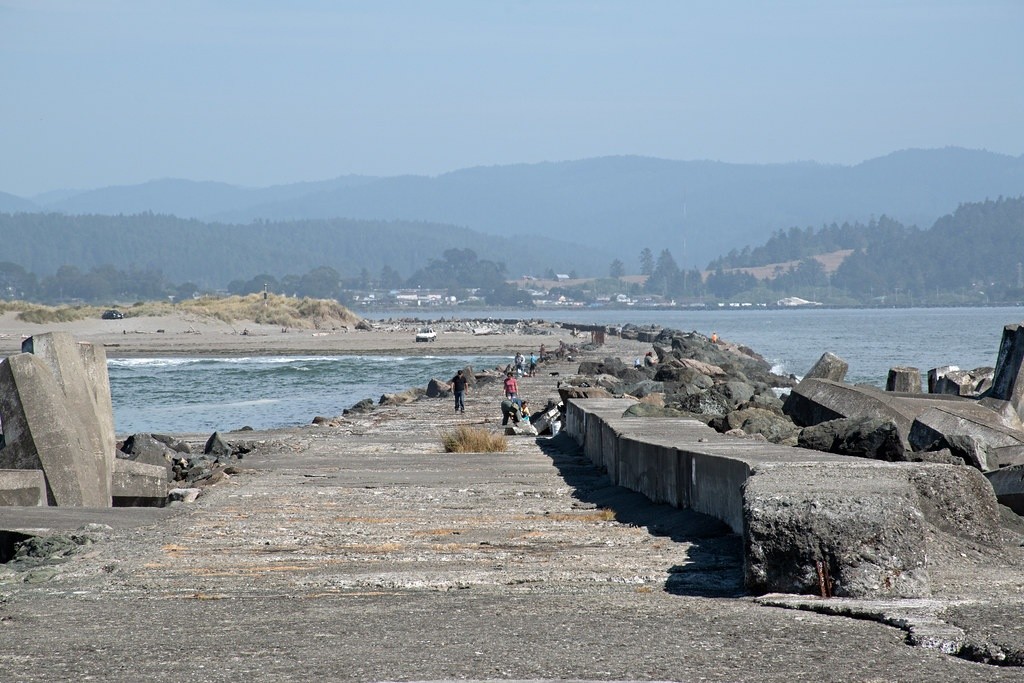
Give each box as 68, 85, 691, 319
501, 398, 530, 427
712, 331, 716, 344
605, 323, 622, 340
634, 352, 658, 370
538, 338, 568, 363
451, 370, 468, 415
515, 351, 538, 378
503, 372, 518, 400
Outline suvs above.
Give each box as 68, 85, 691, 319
416, 327, 437, 342
102, 309, 124, 320
